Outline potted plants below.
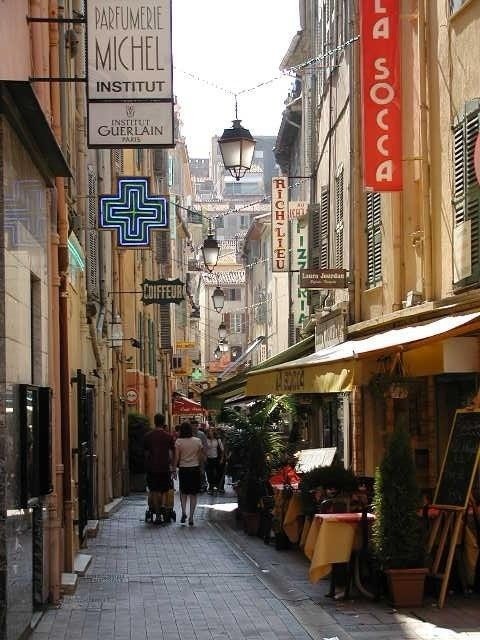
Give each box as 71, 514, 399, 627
367, 429, 435, 611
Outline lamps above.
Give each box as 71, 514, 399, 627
105, 337, 142, 349
217, 313, 230, 343
210, 272, 232, 315
196, 216, 222, 272
216, 91, 258, 182
220, 340, 232, 352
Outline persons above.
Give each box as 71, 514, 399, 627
145, 411, 226, 524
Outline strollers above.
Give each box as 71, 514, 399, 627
146, 469, 177, 524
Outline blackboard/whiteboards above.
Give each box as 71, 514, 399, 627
431, 409, 480, 510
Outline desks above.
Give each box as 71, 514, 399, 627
272, 480, 374, 554
306, 510, 378, 602
